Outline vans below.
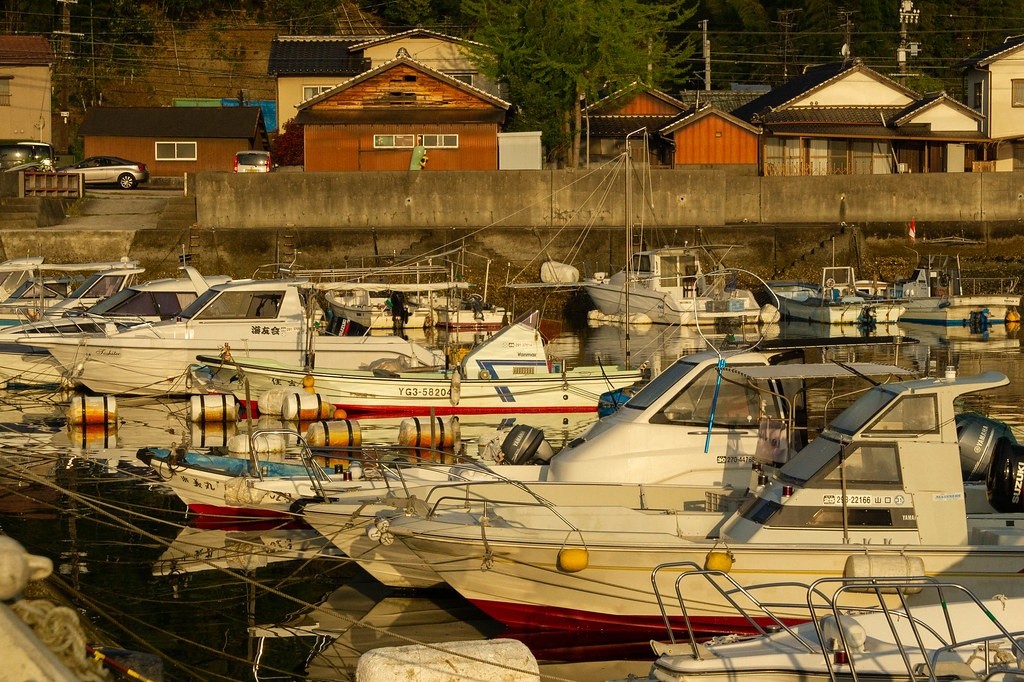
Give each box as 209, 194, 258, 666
0, 142, 52, 171
233, 150, 271, 173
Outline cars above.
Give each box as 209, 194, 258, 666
57, 156, 149, 190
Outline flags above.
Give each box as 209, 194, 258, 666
908, 218, 916, 240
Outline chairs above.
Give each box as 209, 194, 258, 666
682, 265, 698, 297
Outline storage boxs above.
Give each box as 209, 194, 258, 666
725, 299, 744, 312
706, 301, 726, 312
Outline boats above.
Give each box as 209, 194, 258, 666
0, 128, 1024, 682
